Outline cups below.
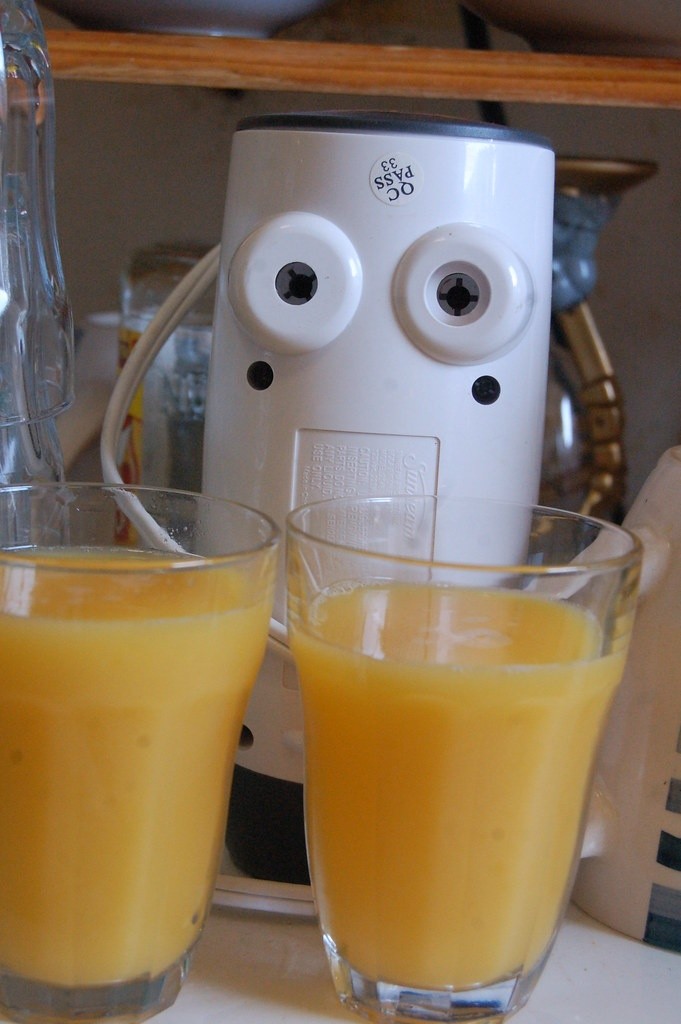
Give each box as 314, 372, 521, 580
91, 311, 121, 367
1, 481, 282, 1024
284, 494, 644, 1024
1, 1, 77, 429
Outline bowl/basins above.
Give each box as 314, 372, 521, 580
456, 0, 680, 60
40, 1, 326, 40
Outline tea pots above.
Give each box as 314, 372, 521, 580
519, 443, 681, 954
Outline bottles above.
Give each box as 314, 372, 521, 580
1, 171, 68, 549
114, 251, 219, 551
